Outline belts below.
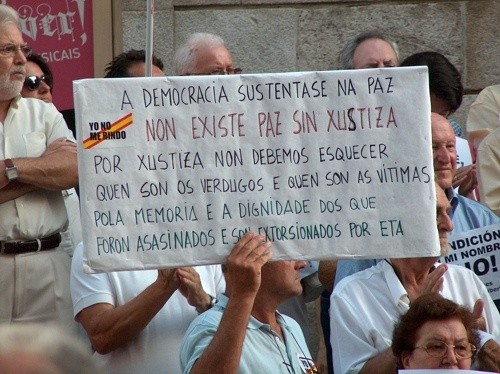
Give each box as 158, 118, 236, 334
0, 232, 61, 254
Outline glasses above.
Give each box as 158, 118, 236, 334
0, 43, 32, 57
187, 67, 243, 75
413, 341, 476, 360
23, 74, 53, 92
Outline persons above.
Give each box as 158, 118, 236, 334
180, 231, 319, 374
0, 322, 95, 374
329, 182, 500, 374
333, 52, 500, 316
319, 30, 462, 374
70, 240, 227, 373
391, 292, 481, 371
0, 6, 78, 324
20, 29, 244, 303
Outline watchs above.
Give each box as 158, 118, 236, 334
4, 159, 19, 183
196, 295, 218, 314
